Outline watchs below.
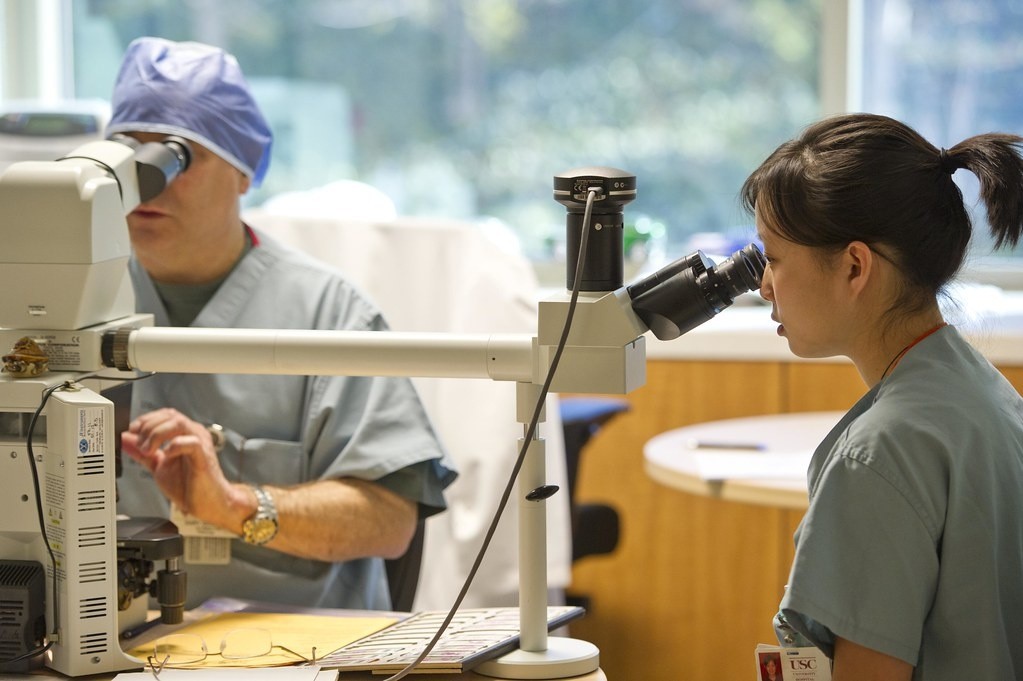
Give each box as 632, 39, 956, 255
239, 484, 281, 547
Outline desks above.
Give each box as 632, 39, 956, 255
641, 410, 858, 513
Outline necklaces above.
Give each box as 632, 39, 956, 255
891, 321, 949, 370
880, 345, 911, 379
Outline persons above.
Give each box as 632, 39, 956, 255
764, 655, 782, 681
741, 112, 1023, 681
102, 36, 460, 612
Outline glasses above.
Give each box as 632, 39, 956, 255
146, 626, 318, 676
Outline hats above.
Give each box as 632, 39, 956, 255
103, 36, 272, 187
